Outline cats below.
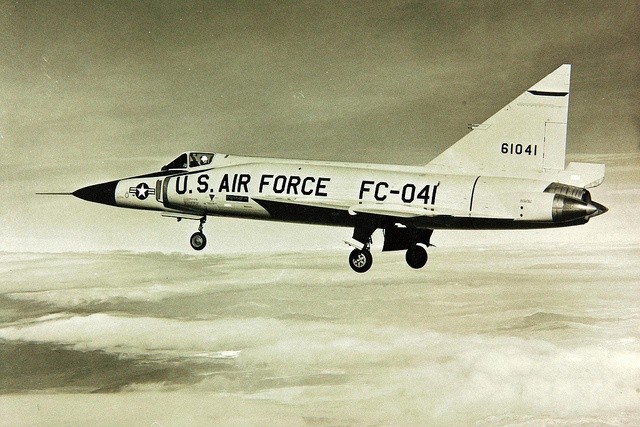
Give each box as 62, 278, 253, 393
34, 64, 608, 272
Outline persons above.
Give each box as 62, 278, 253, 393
200, 155, 208, 165
